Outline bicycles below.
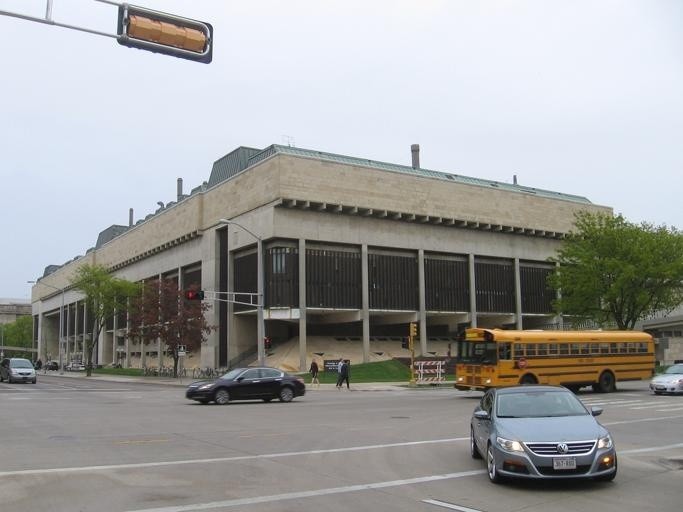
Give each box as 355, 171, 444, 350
142, 365, 230, 378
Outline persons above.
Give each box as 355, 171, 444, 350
336, 359, 351, 392
308, 357, 321, 389
36, 351, 53, 373
335, 358, 344, 388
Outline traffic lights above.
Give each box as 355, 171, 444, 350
409, 322, 416, 337
263, 337, 271, 349
184, 290, 203, 300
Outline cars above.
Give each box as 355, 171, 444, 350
185, 367, 306, 404
0, 357, 35, 384
32, 357, 101, 372
649, 362, 682, 394
467, 384, 617, 483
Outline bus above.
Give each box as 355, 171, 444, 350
454, 328, 655, 393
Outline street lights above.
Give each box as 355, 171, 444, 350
26, 280, 64, 375
219, 217, 266, 366
